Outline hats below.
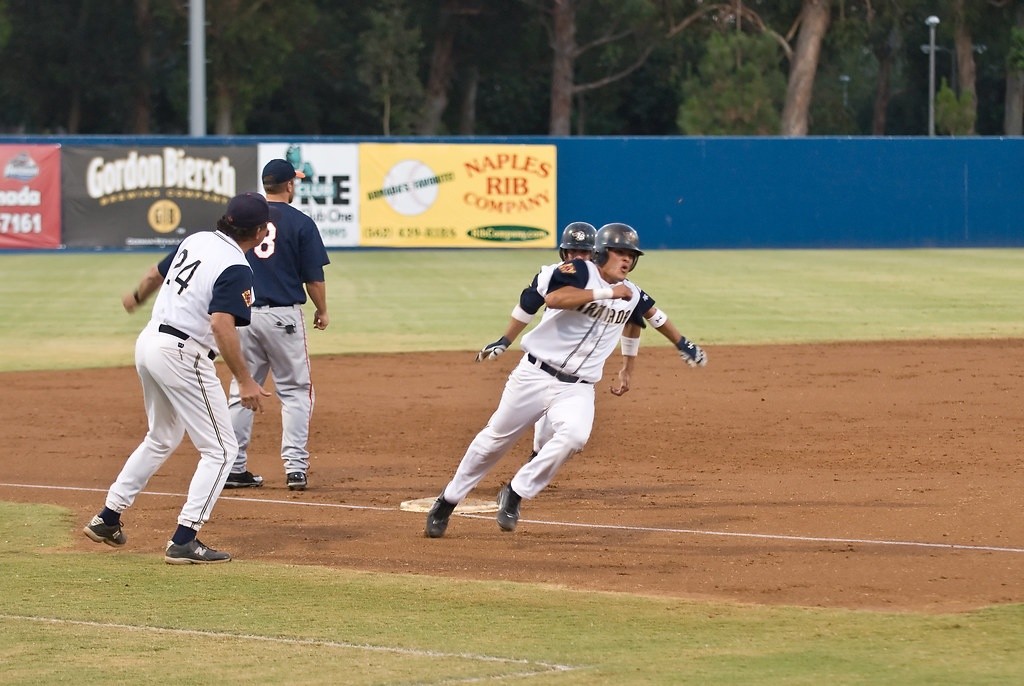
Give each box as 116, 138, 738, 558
225, 192, 273, 231
262, 159, 305, 184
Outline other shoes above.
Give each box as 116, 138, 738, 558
528, 450, 538, 462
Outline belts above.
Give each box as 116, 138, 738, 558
159, 324, 216, 361
528, 353, 588, 384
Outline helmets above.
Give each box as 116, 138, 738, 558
559, 222, 597, 261
591, 223, 645, 272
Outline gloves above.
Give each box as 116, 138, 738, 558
675, 336, 708, 367
474, 336, 512, 362
285, 325, 293, 334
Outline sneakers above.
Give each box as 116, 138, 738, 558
427, 480, 458, 537
284, 472, 307, 486
496, 481, 522, 531
83, 514, 126, 547
164, 536, 231, 564
223, 470, 263, 488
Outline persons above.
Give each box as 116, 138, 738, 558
225, 159, 331, 490
476, 222, 707, 462
425, 223, 646, 538
84, 192, 272, 564
